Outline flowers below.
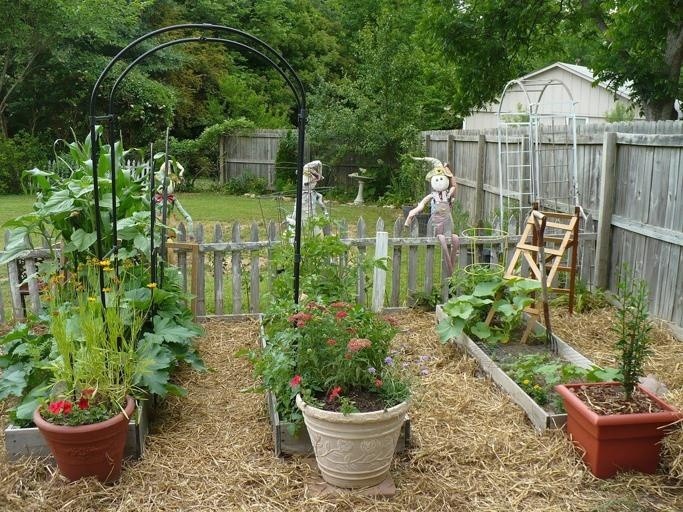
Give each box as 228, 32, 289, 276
36, 259, 155, 421
282, 291, 397, 409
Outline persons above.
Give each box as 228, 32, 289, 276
156, 160, 193, 235
284, 159, 330, 242
403, 153, 459, 278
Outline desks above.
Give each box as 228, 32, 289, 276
348, 174, 376, 205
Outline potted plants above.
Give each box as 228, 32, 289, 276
555, 261, 683, 478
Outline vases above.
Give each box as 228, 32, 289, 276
32, 396, 136, 483
294, 391, 412, 490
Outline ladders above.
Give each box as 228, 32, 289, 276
480, 202, 581, 346
